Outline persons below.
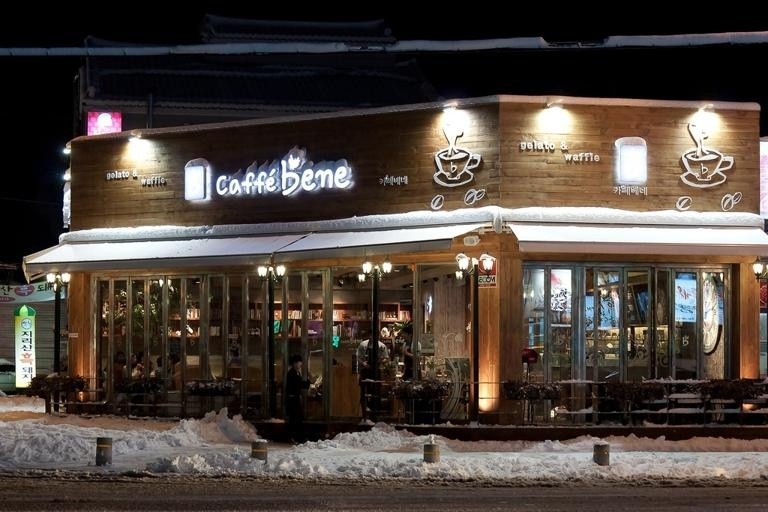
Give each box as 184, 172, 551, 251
287, 357, 316, 414
400, 327, 422, 381
114, 352, 180, 395
356, 330, 390, 413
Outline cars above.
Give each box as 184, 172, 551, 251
1, 362, 17, 393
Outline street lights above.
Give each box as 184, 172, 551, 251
356, 252, 393, 421
257, 254, 287, 418
454, 250, 497, 425
46, 271, 73, 415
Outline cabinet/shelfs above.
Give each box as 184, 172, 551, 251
526, 307, 705, 379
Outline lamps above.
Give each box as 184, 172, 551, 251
614, 136, 648, 185
184, 158, 212, 201
751, 256, 764, 283
